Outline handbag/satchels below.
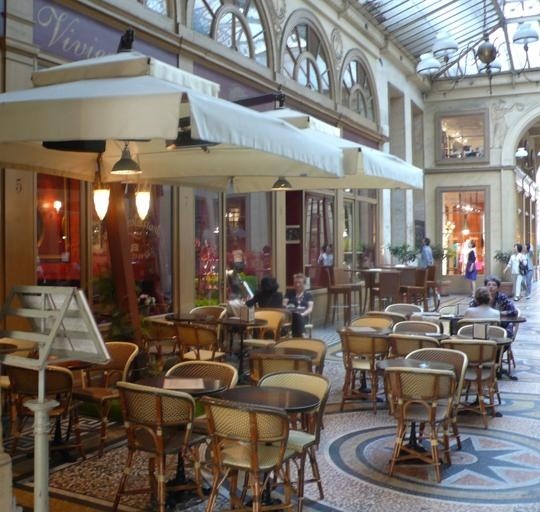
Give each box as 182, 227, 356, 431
519, 259, 529, 276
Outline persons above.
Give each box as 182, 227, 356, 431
464, 286, 500, 326
521, 244, 534, 299
137, 279, 167, 314
318, 244, 334, 266
464, 239, 476, 298
502, 244, 526, 301
469, 277, 518, 363
282, 273, 314, 338
421, 237, 433, 270
246, 277, 287, 339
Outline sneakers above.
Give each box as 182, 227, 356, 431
514, 297, 521, 301
526, 296, 530, 299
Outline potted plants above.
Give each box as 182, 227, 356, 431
495, 251, 513, 298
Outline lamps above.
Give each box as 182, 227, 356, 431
461, 214, 471, 235
93, 170, 112, 223
272, 177, 292, 191
135, 182, 154, 223
112, 142, 143, 177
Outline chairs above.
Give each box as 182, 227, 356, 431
393, 321, 441, 337
164, 360, 238, 468
142, 318, 182, 360
1, 337, 37, 435
1, 360, 88, 462
240, 309, 286, 347
502, 308, 521, 374
366, 311, 406, 324
189, 305, 228, 360
439, 306, 456, 315
257, 370, 331, 512
383, 366, 456, 483
420, 265, 439, 310
363, 271, 384, 313
346, 315, 395, 360
386, 304, 423, 316
457, 324, 508, 405
322, 266, 352, 328
302, 303, 314, 339
388, 333, 439, 357
249, 353, 316, 436
199, 395, 294, 512
257, 308, 293, 341
272, 338, 328, 430
338, 330, 392, 415
442, 340, 497, 429
66, 342, 139, 458
113, 381, 205, 511
400, 268, 429, 311
331, 266, 353, 326
457, 319, 501, 336
405, 347, 468, 450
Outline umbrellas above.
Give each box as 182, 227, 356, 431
150, 106, 424, 194
0, 50, 343, 183
